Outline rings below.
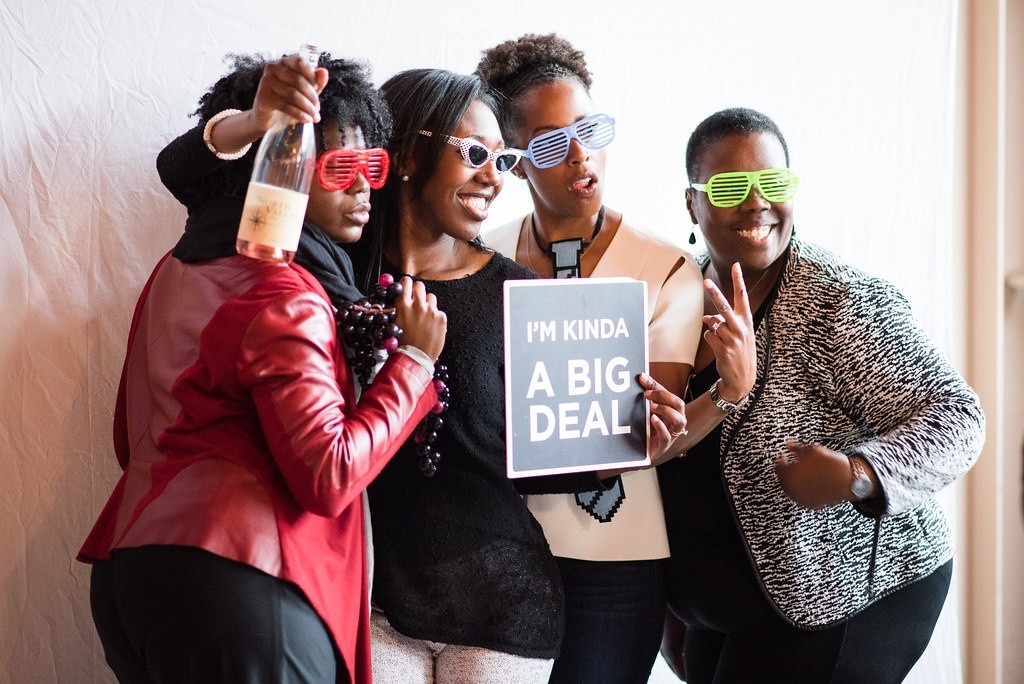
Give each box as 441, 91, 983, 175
668, 426, 688, 437
711, 319, 725, 335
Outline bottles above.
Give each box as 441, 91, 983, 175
237, 44, 322, 265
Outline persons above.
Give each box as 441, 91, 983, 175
74, 31, 984, 684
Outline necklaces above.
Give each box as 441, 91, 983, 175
526, 212, 598, 276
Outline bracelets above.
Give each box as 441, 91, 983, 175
202, 109, 252, 160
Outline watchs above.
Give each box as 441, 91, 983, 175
847, 456, 874, 502
708, 378, 750, 413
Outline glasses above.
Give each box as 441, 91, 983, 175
505, 113, 616, 169
417, 130, 521, 174
691, 167, 799, 208
316, 149, 390, 192
